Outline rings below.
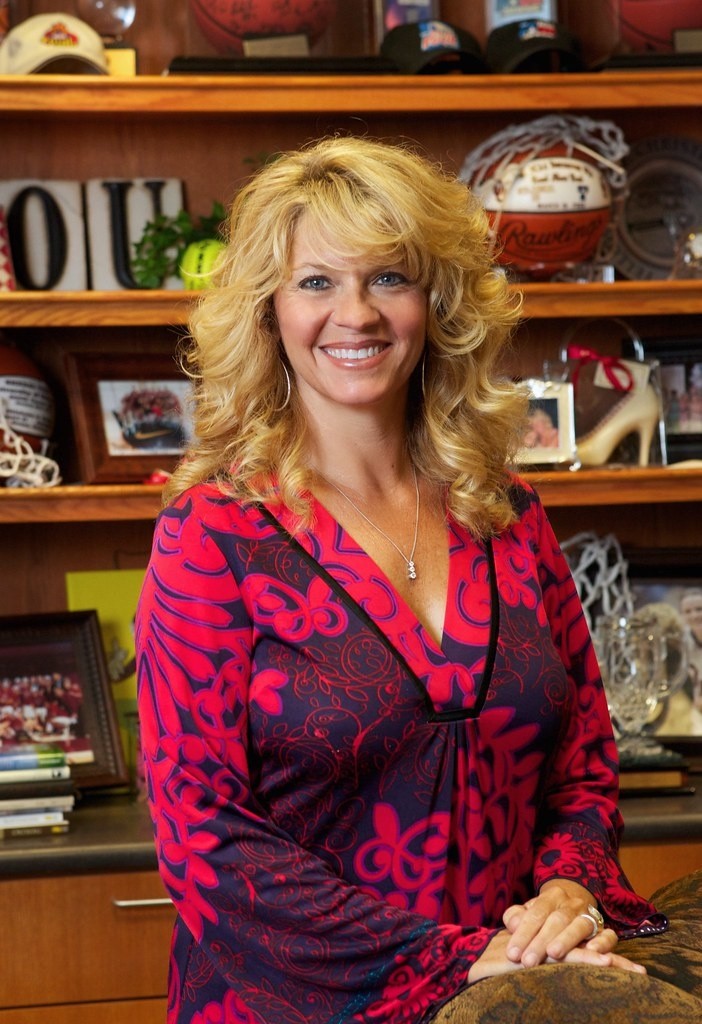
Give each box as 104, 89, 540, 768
578, 914, 597, 939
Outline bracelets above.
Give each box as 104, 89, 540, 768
587, 904, 605, 928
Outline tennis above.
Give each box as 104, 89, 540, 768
180, 237, 230, 289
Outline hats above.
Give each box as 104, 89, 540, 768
1, 12, 107, 75
483, 19, 580, 75
381, 19, 483, 77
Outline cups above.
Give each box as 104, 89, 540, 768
593, 613, 690, 768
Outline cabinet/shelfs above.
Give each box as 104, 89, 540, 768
0, 58, 702, 1024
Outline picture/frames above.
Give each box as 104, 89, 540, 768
617, 333, 702, 465
0, 606, 133, 798
505, 379, 576, 466
60, 351, 204, 485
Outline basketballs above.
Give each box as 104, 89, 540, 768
608, 0, 702, 57
0, 343, 53, 482
462, 124, 614, 275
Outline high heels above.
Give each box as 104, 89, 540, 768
555, 383, 657, 466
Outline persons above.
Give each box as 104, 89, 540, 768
135, 141, 671, 1024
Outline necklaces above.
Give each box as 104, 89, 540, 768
308, 460, 419, 579
